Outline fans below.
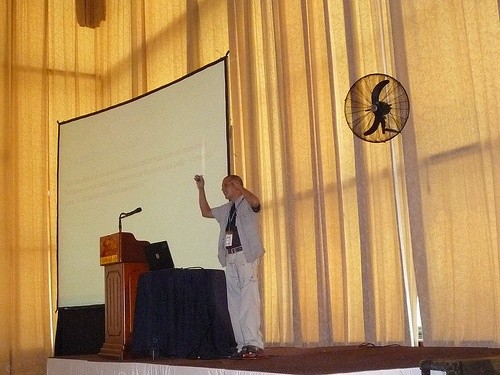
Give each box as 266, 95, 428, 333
344, 72, 409, 143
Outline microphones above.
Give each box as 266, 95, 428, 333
121, 207, 142, 218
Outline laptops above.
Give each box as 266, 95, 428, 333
145, 241, 188, 271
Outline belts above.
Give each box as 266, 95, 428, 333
228, 246, 243, 254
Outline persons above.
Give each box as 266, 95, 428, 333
195, 174, 265, 358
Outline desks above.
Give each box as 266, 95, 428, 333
134, 268, 236, 359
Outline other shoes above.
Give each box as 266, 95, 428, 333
246, 345, 265, 359
230, 346, 247, 359
